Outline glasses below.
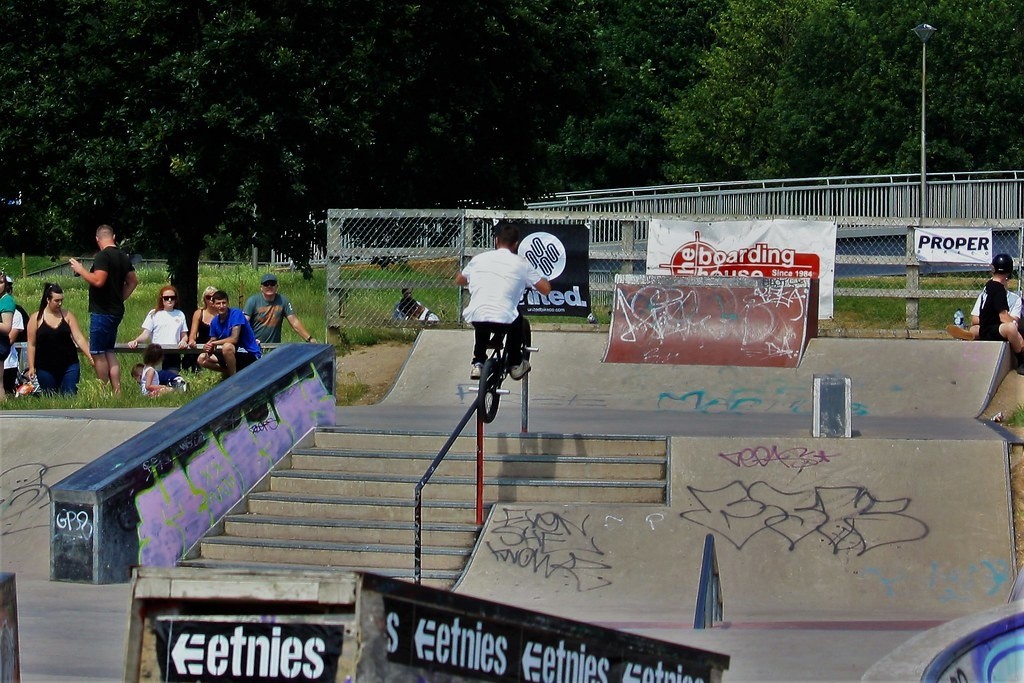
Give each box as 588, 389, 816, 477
260, 281, 277, 287
162, 296, 176, 301
204, 294, 213, 300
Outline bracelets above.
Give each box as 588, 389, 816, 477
89, 358, 94, 362
306, 336, 312, 342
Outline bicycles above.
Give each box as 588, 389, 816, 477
467, 286, 540, 424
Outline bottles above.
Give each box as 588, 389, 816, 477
953, 308, 964, 341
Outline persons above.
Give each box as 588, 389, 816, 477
187, 285, 218, 371
0, 270, 31, 403
242, 275, 317, 345
399, 287, 422, 319
453, 224, 552, 381
141, 344, 173, 397
69, 225, 137, 398
943, 253, 1024, 373
131, 363, 186, 393
197, 289, 263, 377
128, 286, 189, 372
27, 282, 95, 399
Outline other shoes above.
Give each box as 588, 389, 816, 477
1016, 349, 1024, 375
946, 324, 974, 340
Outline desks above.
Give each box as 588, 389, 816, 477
14, 342, 254, 382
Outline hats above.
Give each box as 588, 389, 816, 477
261, 274, 276, 283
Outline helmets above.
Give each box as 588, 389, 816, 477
991, 254, 1013, 275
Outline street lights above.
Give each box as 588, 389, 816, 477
910, 23, 939, 230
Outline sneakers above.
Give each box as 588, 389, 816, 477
510, 359, 531, 380
470, 363, 484, 379
